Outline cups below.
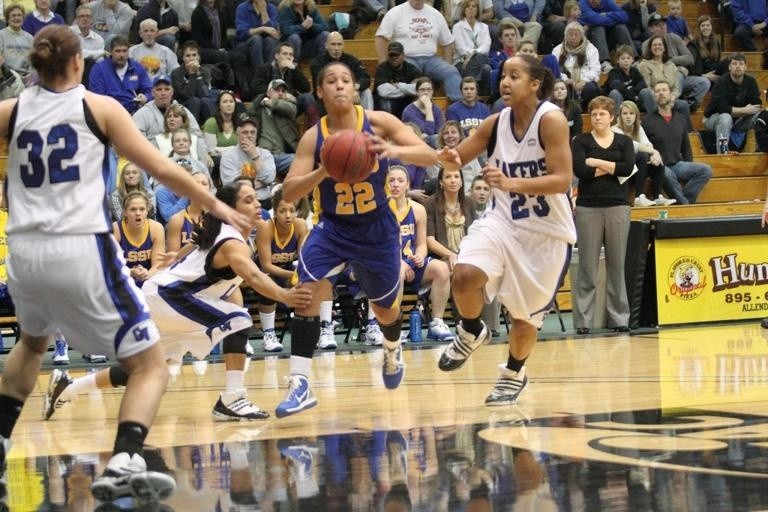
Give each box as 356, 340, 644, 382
718, 137, 729, 154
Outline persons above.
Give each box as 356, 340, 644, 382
0, 0, 768, 504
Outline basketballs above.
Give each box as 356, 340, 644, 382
322, 131, 376, 185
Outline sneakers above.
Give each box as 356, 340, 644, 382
427, 318, 454, 342
633, 194, 656, 207
316, 321, 339, 350
654, 194, 676, 207
383, 346, 405, 389
438, 319, 492, 371
92, 451, 176, 506
484, 364, 529, 405
246, 340, 256, 355
212, 390, 270, 421
83, 353, 108, 363
42, 369, 74, 421
275, 375, 317, 418
262, 331, 283, 351
52, 341, 70, 364
364, 324, 384, 347
0, 436, 13, 512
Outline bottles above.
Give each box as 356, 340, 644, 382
410, 312, 422, 342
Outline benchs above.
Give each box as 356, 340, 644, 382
310, 0, 492, 120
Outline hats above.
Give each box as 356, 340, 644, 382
236, 113, 257, 125
388, 42, 403, 56
267, 79, 289, 90
648, 14, 668, 26
153, 75, 172, 86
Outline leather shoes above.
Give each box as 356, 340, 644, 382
615, 326, 631, 332
577, 327, 590, 335
